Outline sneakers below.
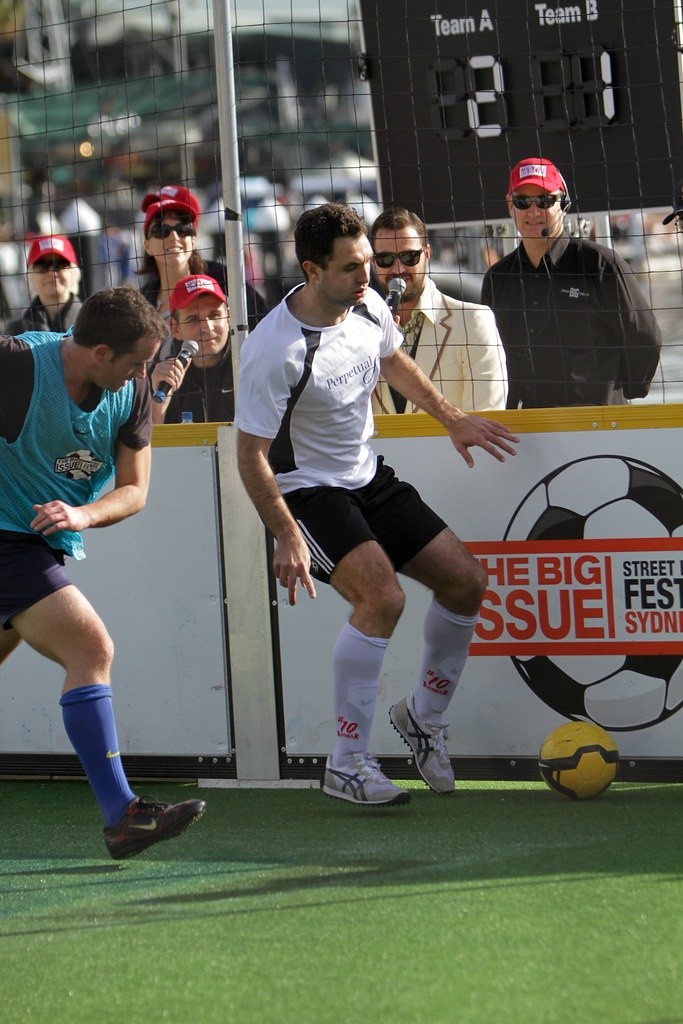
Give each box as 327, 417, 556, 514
389, 696, 457, 794
322, 752, 412, 808
102, 795, 205, 860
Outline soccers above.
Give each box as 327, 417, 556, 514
537, 720, 620, 801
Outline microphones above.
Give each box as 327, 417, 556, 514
540, 204, 569, 236
152, 339, 200, 403
386, 278, 406, 319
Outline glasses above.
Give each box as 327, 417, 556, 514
32, 260, 71, 273
512, 195, 562, 210
147, 222, 195, 242
373, 244, 426, 269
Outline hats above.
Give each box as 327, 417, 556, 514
26, 235, 78, 264
170, 275, 229, 314
509, 158, 565, 194
140, 185, 199, 225
144, 200, 196, 232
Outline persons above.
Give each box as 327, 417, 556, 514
0, 286, 204, 858
232, 204, 519, 810
480, 158, 661, 411
369, 208, 508, 414
0, 186, 272, 424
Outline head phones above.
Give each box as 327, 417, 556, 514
559, 173, 572, 212
